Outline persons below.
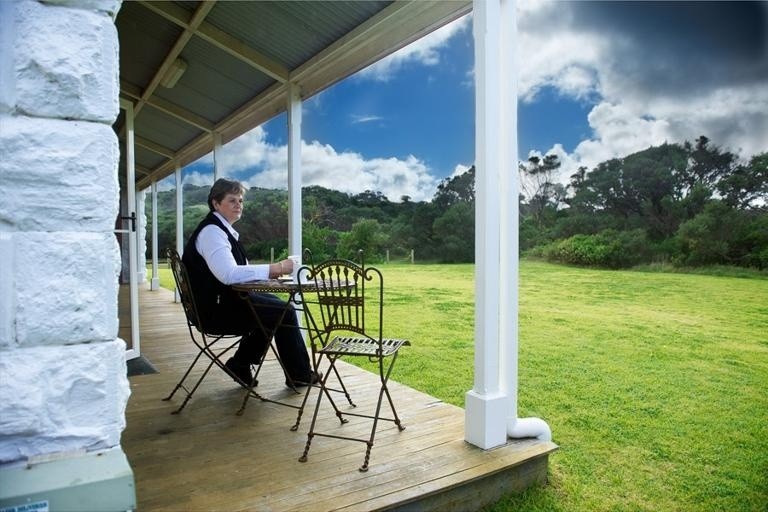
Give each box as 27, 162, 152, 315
182, 179, 323, 388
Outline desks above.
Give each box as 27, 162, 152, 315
232, 268, 358, 429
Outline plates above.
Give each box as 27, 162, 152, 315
277, 277, 294, 281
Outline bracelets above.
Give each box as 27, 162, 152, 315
279, 262, 283, 274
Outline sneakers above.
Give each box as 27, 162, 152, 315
225, 357, 259, 387
285, 369, 323, 386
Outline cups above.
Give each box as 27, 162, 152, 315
288, 254, 301, 276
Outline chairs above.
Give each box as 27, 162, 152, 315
159, 247, 299, 413
297, 259, 414, 473
